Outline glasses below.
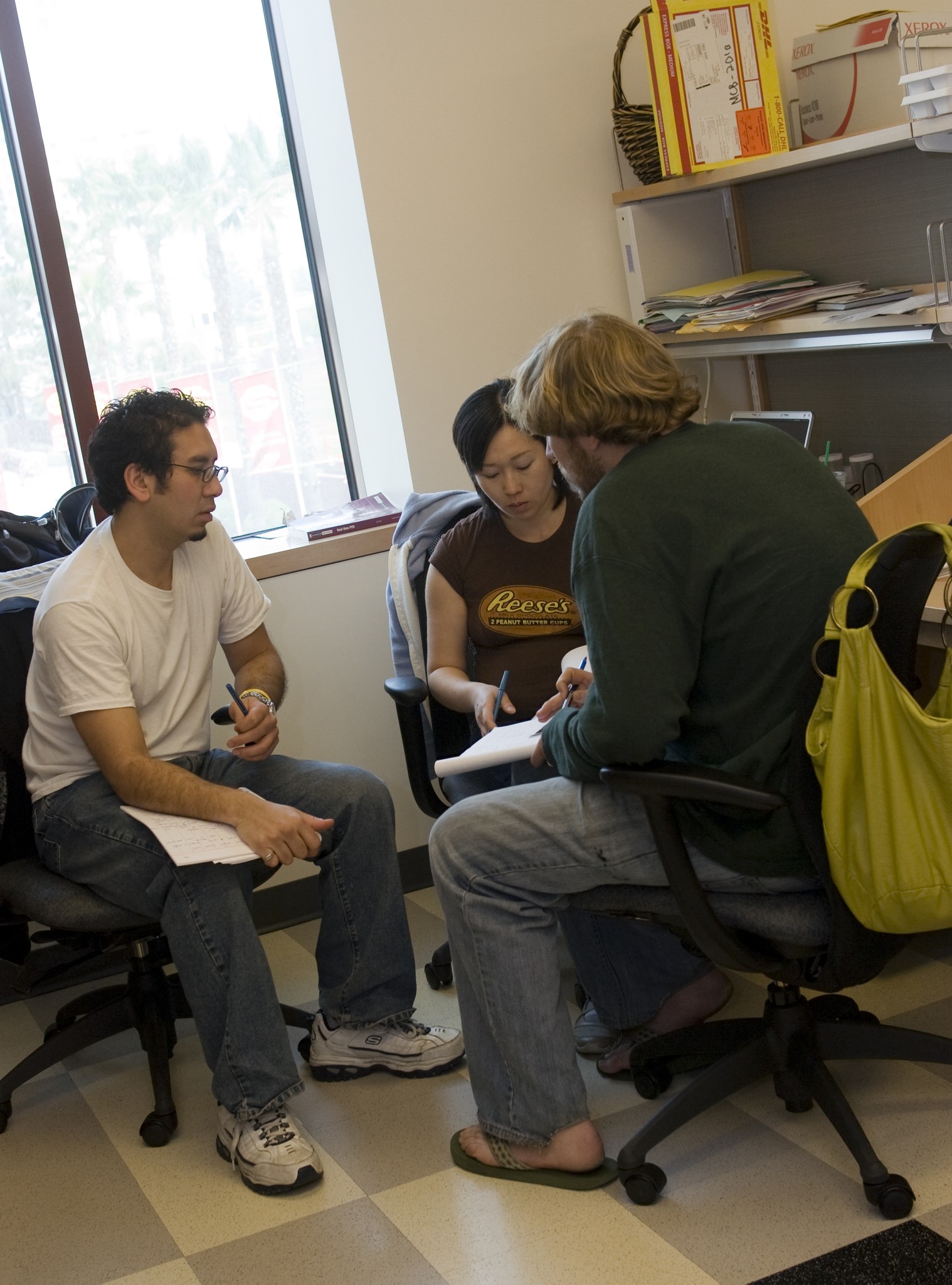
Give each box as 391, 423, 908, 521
163, 463, 228, 484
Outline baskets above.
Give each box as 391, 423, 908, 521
612, 5, 663, 185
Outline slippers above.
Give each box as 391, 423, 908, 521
450, 1124, 619, 1192
596, 983, 735, 1081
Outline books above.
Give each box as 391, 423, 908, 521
817, 287, 914, 311
434, 713, 559, 778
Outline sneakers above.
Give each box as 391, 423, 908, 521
308, 1009, 468, 1081
214, 1099, 322, 1197
573, 996, 621, 1054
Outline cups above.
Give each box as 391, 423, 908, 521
832, 471, 847, 491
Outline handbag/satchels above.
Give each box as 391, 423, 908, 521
804, 520, 952, 933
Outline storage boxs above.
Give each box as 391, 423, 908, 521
790, 11, 952, 144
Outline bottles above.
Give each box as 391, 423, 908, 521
819, 453, 844, 472
849, 453, 877, 502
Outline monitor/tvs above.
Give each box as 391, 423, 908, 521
729, 410, 815, 451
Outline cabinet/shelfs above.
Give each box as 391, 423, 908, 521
609, 117, 952, 655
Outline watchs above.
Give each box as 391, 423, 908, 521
242, 691, 276, 717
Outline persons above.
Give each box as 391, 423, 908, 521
453, 315, 874, 1193
429, 377, 620, 1062
23, 388, 461, 1192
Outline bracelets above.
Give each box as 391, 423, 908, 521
239, 689, 271, 701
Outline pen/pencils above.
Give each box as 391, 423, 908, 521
488, 671, 509, 734
562, 656, 587, 710
226, 684, 249, 716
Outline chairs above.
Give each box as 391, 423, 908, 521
0, 594, 316, 1143
381, 494, 492, 987
551, 522, 952, 1212
